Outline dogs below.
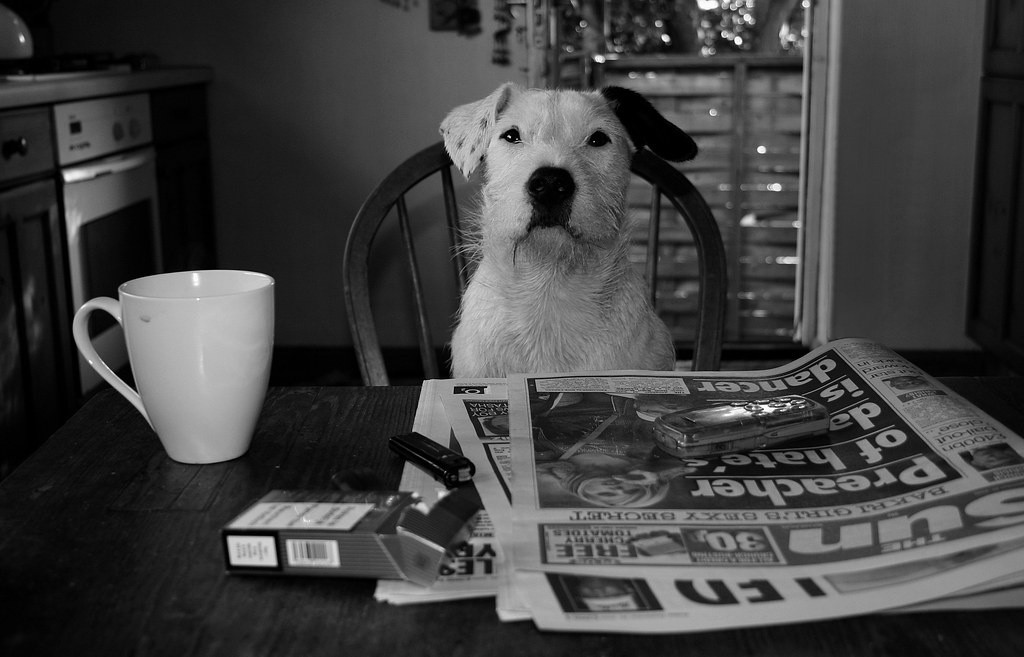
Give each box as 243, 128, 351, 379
440, 81, 698, 379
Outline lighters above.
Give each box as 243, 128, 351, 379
387, 430, 475, 483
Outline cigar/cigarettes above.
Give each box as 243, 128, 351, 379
220, 483, 484, 581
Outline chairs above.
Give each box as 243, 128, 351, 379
341, 140, 730, 388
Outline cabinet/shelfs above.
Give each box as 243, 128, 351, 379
0, 105, 78, 480
149, 82, 217, 272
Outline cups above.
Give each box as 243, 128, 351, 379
71, 269, 278, 462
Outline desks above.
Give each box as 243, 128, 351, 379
0, 382, 1024, 657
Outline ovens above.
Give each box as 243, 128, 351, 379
52, 92, 164, 395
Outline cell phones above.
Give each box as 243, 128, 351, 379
651, 395, 830, 458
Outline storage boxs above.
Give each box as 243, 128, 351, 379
219, 488, 483, 588
